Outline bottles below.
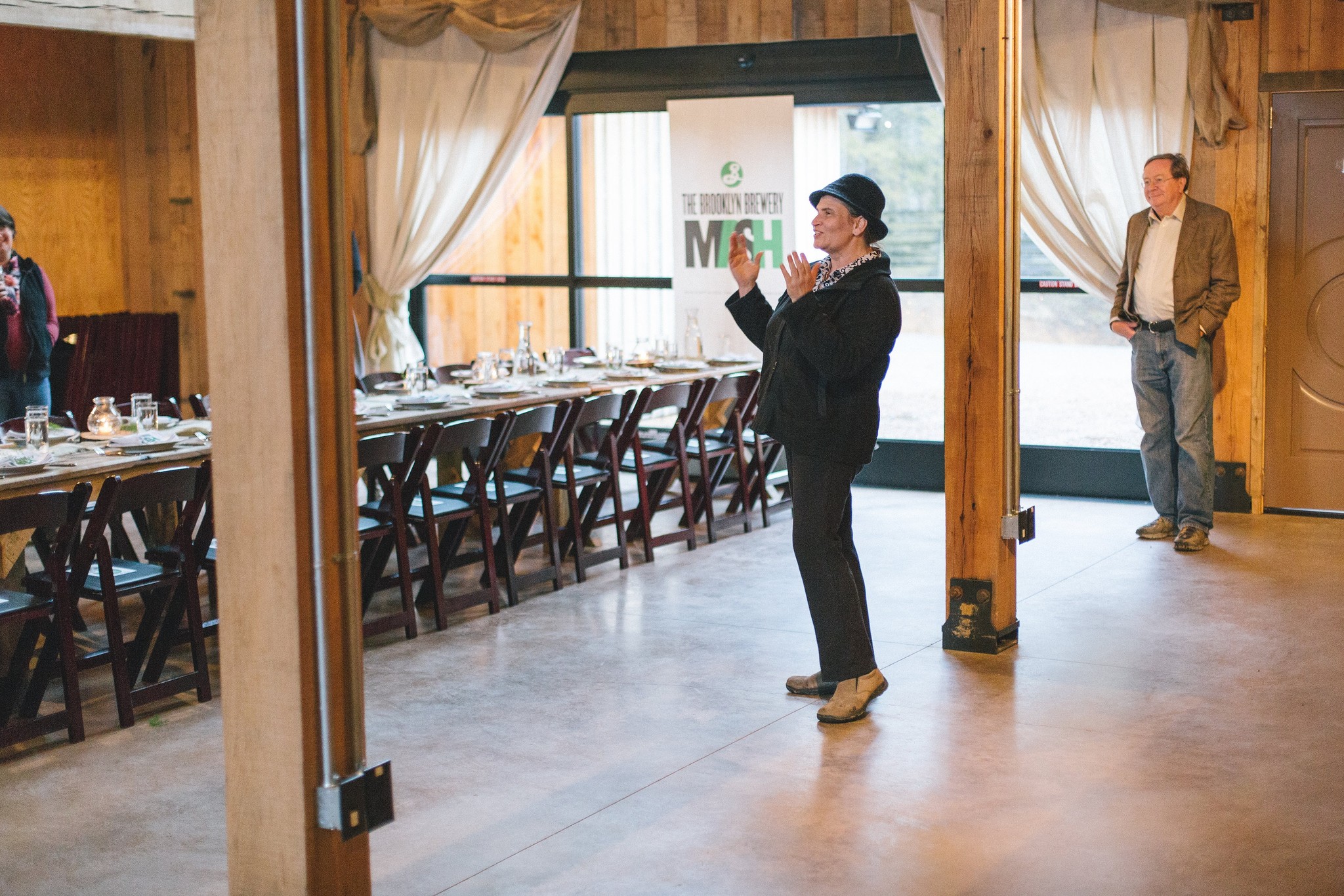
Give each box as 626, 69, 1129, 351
685, 307, 703, 359
87, 396, 122, 436
515, 321, 537, 378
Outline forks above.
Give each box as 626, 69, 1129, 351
195, 432, 207, 441
94, 446, 141, 456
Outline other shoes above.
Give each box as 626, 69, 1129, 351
818, 662, 888, 723
785, 671, 838, 695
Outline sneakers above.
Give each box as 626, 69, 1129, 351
1135, 517, 1179, 539
1173, 525, 1209, 551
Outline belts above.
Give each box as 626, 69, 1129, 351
1139, 321, 1178, 334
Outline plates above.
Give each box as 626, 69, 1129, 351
450, 370, 510, 383
607, 374, 648, 382
354, 406, 377, 418
106, 436, 189, 454
3, 428, 77, 445
0, 455, 57, 476
625, 362, 655, 368
571, 356, 607, 368
373, 379, 436, 394
708, 360, 752, 368
653, 364, 704, 373
546, 377, 594, 388
474, 385, 531, 400
396, 396, 449, 410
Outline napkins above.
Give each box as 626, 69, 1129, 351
110, 429, 180, 445
0, 447, 55, 468
5, 430, 27, 439
395, 392, 451, 403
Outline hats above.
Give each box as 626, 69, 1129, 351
809, 174, 888, 243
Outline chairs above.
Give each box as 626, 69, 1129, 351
0, 346, 793, 756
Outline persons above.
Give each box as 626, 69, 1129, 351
723, 174, 902, 723
1109, 153, 1240, 551
0, 207, 59, 424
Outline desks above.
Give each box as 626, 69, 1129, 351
0, 385, 592, 676
463, 363, 783, 549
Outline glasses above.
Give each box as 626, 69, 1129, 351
1141, 175, 1178, 188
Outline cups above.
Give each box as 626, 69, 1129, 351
656, 338, 668, 362
607, 343, 624, 371
471, 351, 499, 383
496, 348, 515, 380
546, 350, 564, 375
24, 405, 50, 455
132, 393, 159, 431
405, 362, 428, 397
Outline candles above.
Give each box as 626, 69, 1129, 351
99, 422, 112, 434
